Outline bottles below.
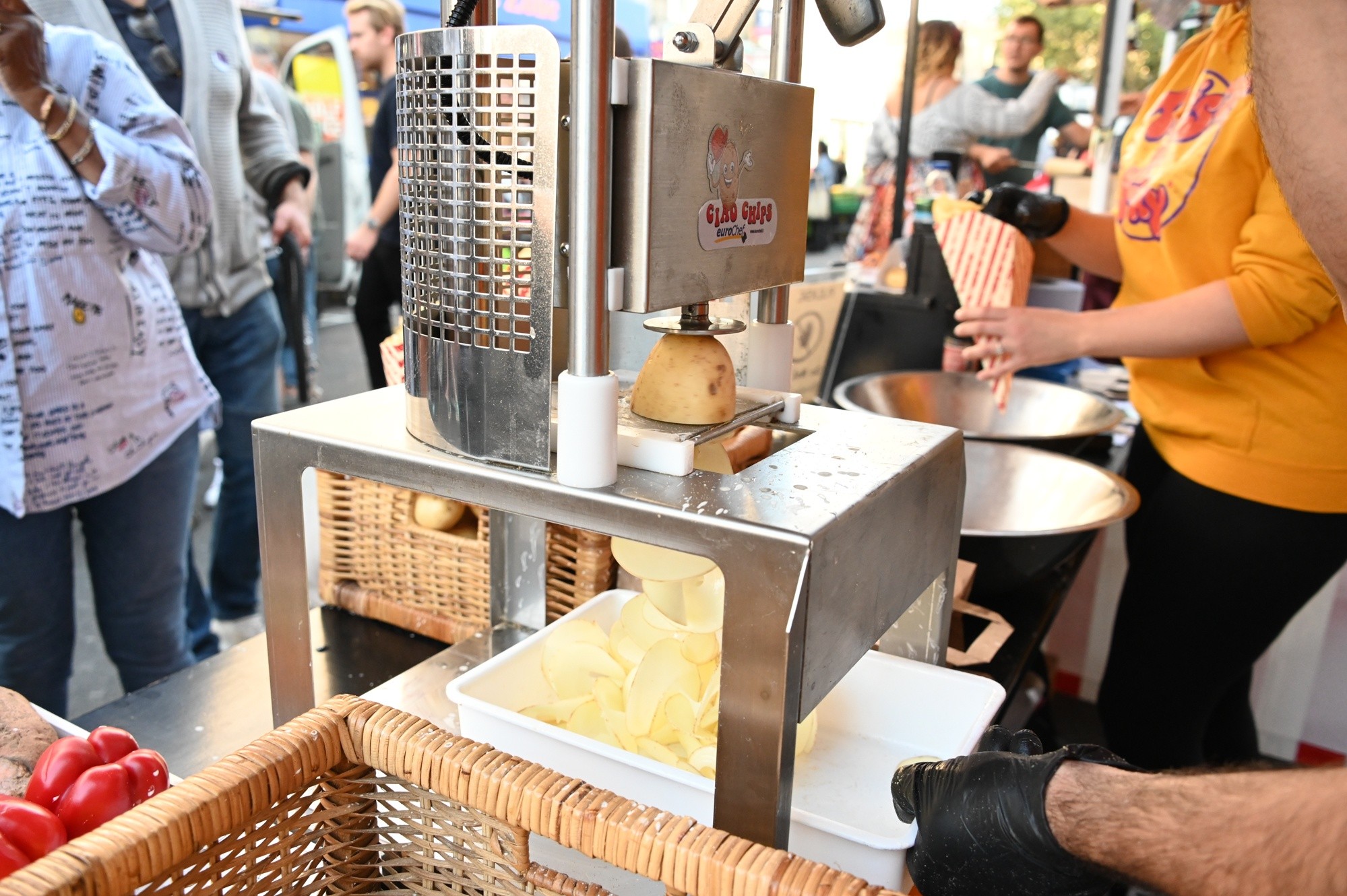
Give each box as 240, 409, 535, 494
924, 160, 957, 200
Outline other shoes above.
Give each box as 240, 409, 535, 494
208, 613, 264, 654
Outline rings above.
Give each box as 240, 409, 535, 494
994, 342, 1002, 359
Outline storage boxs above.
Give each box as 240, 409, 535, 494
448, 587, 1006, 896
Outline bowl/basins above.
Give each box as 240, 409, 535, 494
958, 440, 1140, 579
830, 368, 1126, 455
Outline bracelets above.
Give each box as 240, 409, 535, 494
48, 93, 79, 141
366, 220, 380, 232
34, 88, 59, 129
70, 134, 96, 168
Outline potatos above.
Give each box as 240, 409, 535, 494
507, 445, 816, 786
414, 492, 481, 542
629, 328, 736, 427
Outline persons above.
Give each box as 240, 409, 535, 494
812, 141, 848, 249
1245, 0, 1346, 322
890, 723, 1346, 896
947, 4, 1343, 770
967, 17, 1145, 195
858, 19, 1068, 255
0, 0, 423, 733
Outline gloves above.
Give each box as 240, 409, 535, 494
890, 725, 1152, 896
962, 181, 1070, 240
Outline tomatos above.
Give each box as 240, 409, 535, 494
0, 725, 173, 881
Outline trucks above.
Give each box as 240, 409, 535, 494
275, 26, 373, 293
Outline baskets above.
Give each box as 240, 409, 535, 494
317, 466, 619, 647
0, 692, 906, 896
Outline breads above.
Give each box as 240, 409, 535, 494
0, 684, 59, 799
